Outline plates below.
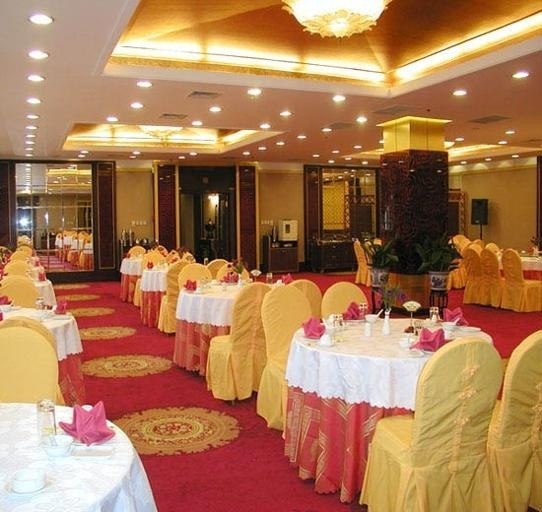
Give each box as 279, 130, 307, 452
4, 479, 54, 498
71, 446, 114, 458
458, 326, 480, 332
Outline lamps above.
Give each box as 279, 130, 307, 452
280, 0, 393, 39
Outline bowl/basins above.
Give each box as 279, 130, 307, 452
364, 314, 378, 323
1, 304, 12, 312
41, 435, 74, 455
13, 466, 45, 487
443, 322, 456, 331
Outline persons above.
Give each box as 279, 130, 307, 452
204, 217, 215, 239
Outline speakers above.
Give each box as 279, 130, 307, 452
470, 198, 489, 224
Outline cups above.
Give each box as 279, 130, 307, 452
192, 270, 275, 294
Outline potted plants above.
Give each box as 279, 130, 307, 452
413, 223, 463, 291
359, 234, 397, 290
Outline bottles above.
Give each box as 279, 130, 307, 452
358, 302, 367, 324
429, 306, 438, 321
319, 312, 344, 346
33, 297, 57, 314
37, 400, 56, 439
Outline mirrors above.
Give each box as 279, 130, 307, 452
11, 159, 97, 282
320, 167, 381, 240
